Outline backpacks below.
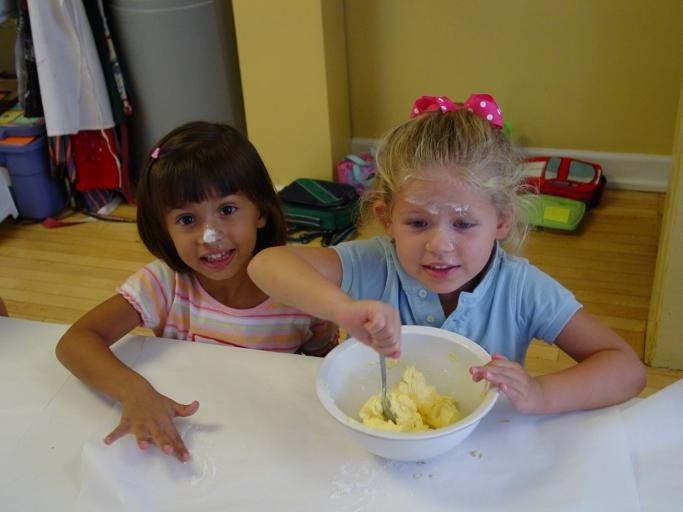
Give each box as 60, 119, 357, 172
277, 178, 362, 246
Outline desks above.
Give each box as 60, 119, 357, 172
0, 314, 682, 511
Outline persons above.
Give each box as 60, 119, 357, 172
247, 93, 647, 414
55, 122, 340, 462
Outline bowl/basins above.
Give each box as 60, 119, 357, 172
313, 324, 506, 462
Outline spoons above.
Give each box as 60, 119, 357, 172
379, 352, 399, 426
